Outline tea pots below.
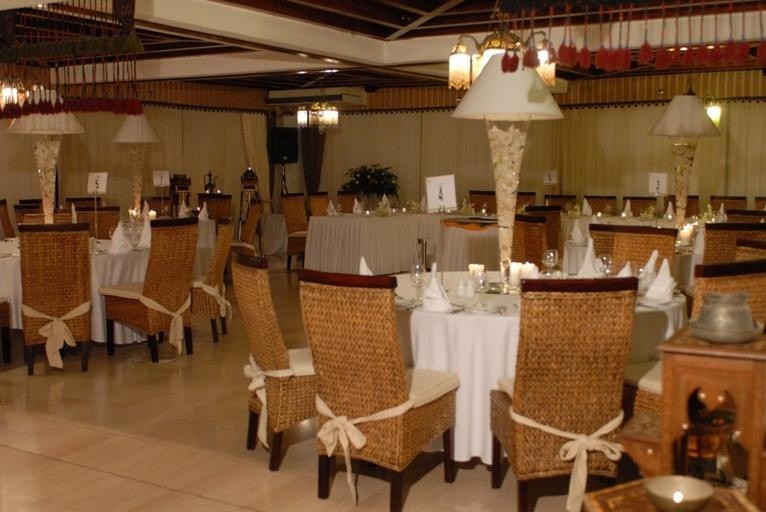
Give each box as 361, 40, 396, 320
201, 170, 218, 193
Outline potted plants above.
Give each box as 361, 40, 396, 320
338, 162, 402, 212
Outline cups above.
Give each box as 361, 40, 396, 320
593, 207, 726, 225
333, 197, 489, 218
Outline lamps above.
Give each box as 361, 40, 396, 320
108, 102, 164, 207
293, 82, 343, 132
446, 0, 566, 96
446, 52, 564, 281
646, 90, 722, 224
4, 86, 87, 223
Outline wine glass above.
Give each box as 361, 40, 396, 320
409, 248, 649, 313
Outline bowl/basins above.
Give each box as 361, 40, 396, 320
643, 474, 715, 511
